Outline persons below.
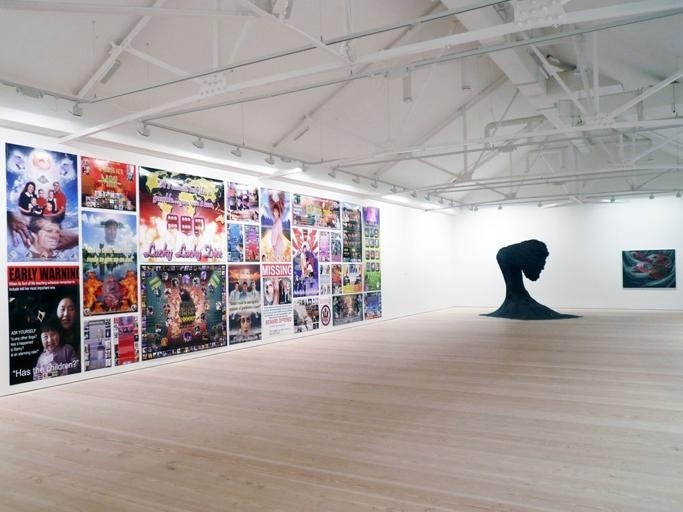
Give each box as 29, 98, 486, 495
228, 281, 260, 301
264, 277, 290, 305
237, 313, 255, 337
33, 297, 79, 380
271, 204, 285, 257
293, 245, 316, 297
7, 181, 78, 258
